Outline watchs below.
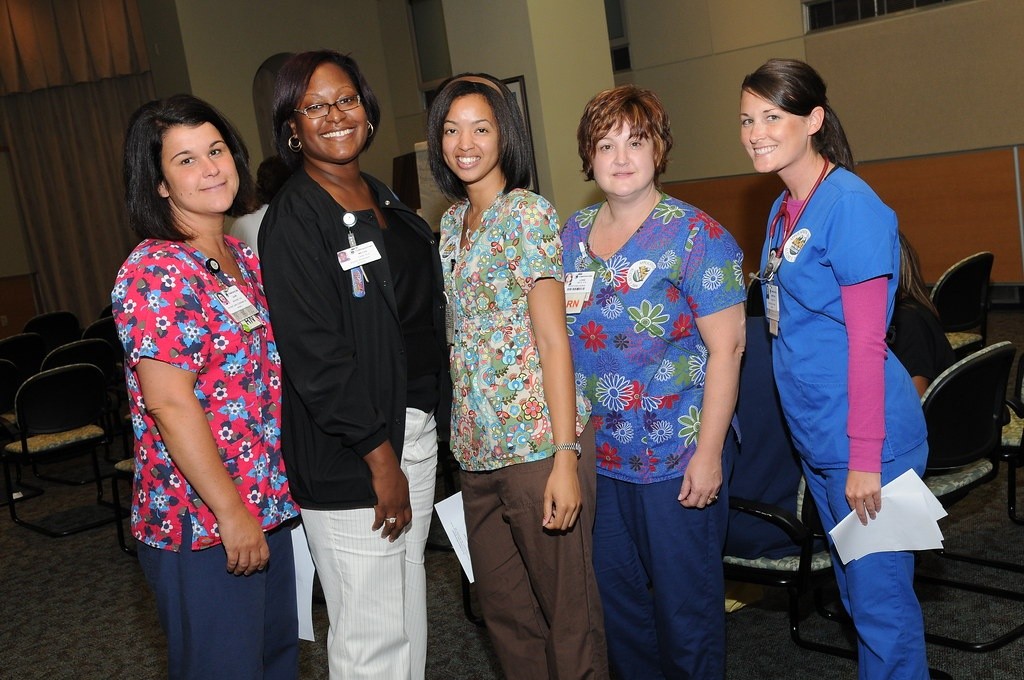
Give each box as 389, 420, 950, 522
552, 441, 582, 458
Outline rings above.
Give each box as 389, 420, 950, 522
384, 517, 397, 523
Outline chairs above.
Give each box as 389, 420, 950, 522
727, 475, 953, 680
0, 303, 138, 560
922, 339, 1024, 574
930, 250, 994, 349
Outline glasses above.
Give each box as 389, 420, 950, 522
293, 94, 362, 119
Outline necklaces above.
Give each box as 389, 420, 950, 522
464, 203, 490, 243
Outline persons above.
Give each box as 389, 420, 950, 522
565, 273, 573, 286
228, 160, 326, 607
886, 232, 955, 398
111, 94, 303, 679
217, 293, 230, 306
739, 57, 928, 680
426, 72, 609, 680
338, 252, 351, 263
560, 85, 748, 680
258, 47, 450, 680
